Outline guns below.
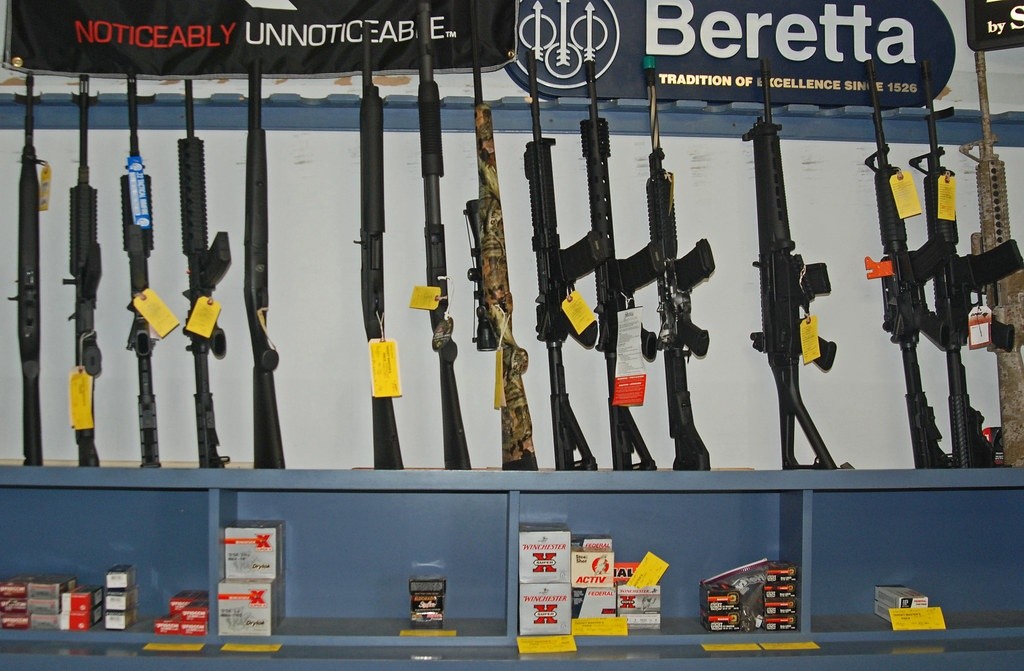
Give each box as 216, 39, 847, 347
351, 20, 407, 470
521, 44, 719, 476
60, 73, 105, 468
458, 1, 540, 472
10, 68, 49, 469
116, 69, 162, 469
739, 57, 842, 471
241, 53, 291, 469
413, 0, 476, 468
862, 46, 1024, 471
176, 77, 235, 467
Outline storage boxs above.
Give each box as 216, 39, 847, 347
409, 577, 447, 626
700, 561, 797, 631
0, 564, 137, 630
153, 589, 209, 637
874, 585, 930, 626
519, 523, 662, 636
216, 520, 286, 637
